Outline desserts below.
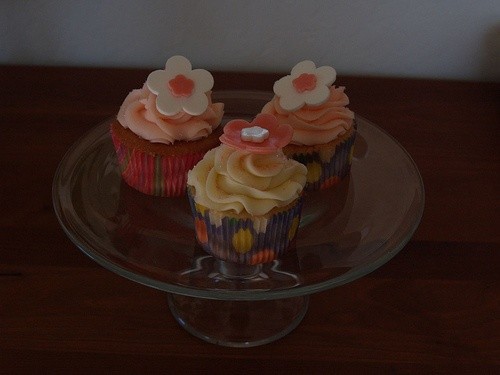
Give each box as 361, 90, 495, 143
258, 59, 358, 193
186, 113, 309, 265
109, 55, 226, 197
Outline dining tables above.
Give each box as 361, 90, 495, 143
1, 62, 500, 375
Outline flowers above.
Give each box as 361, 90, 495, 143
274, 60, 337, 111
219, 111, 293, 154
147, 56, 214, 116
